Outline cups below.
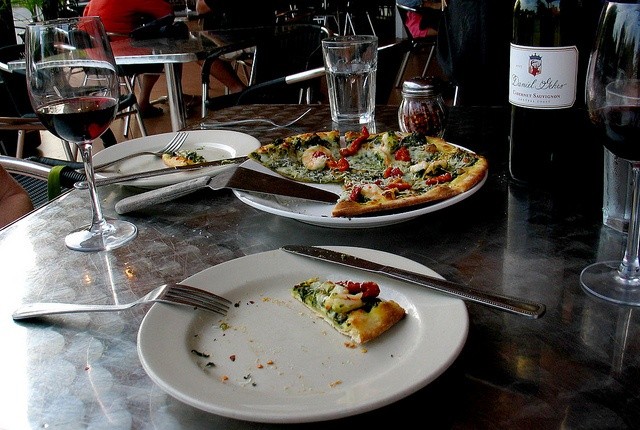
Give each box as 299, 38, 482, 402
321, 34, 380, 127
601, 79, 639, 236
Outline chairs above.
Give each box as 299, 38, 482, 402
200, 24, 331, 118
394, 2, 440, 88
0, 154, 90, 232
233, 38, 413, 104
1, 111, 48, 160
0, 41, 136, 160
76, 44, 147, 137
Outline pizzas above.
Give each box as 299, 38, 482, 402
248, 125, 489, 216
290, 276, 407, 345
161, 150, 207, 167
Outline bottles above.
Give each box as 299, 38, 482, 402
509, 0, 581, 190
399, 78, 448, 140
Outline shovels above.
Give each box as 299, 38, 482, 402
114, 166, 339, 215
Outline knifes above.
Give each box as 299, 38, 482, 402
73, 157, 250, 189
282, 244, 548, 320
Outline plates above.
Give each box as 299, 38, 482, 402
136, 244, 470, 423
233, 142, 489, 229
89, 129, 263, 189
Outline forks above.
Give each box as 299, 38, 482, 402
75, 132, 190, 174
199, 108, 316, 130
13, 283, 234, 320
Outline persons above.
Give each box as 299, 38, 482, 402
0, 164, 34, 229
196, 0, 327, 105
396, 0, 448, 81
75, 0, 203, 119
438, 0, 513, 105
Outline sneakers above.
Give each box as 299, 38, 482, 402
138, 103, 164, 119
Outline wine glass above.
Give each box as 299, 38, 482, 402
25, 14, 141, 253
580, 2, 640, 307
